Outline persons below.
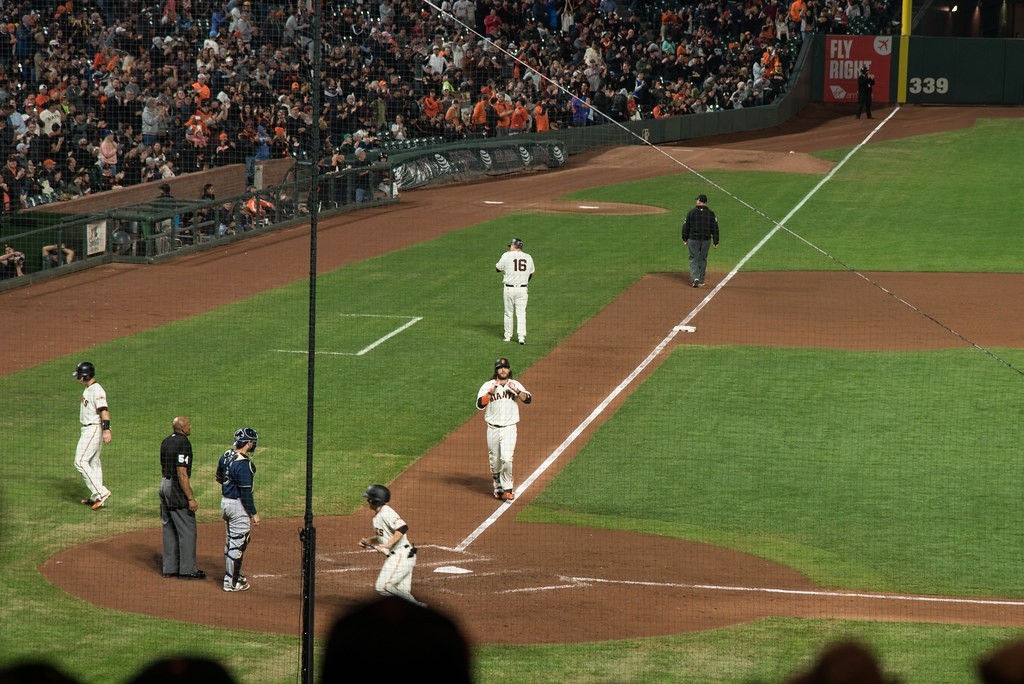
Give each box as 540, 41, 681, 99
856, 66, 876, 120
0, 0, 890, 244
682, 194, 719, 287
215, 428, 260, 591
358, 485, 428, 607
0, 243, 29, 280
475, 358, 531, 500
496, 238, 535, 345
158, 416, 206, 580
72, 362, 112, 510
42, 243, 74, 270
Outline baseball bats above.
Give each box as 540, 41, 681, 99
361, 541, 390, 556
